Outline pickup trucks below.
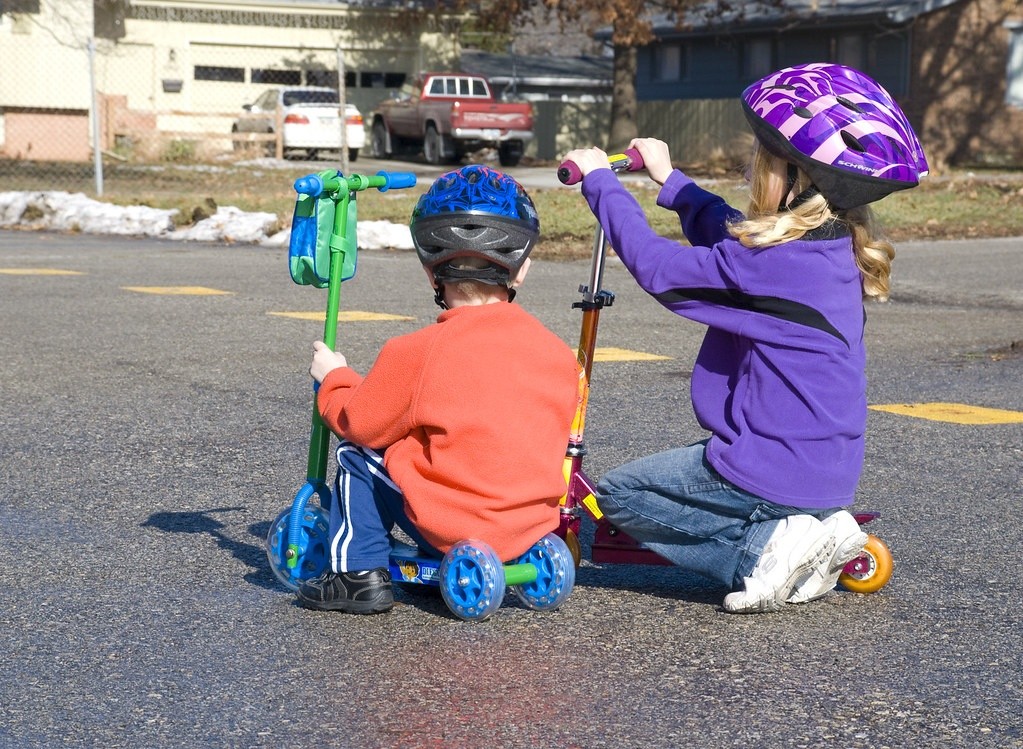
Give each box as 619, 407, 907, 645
366, 67, 537, 167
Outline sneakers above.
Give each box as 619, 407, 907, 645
786, 509, 868, 603
723, 514, 836, 613
297, 569, 394, 613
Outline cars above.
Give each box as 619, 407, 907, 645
231, 85, 366, 162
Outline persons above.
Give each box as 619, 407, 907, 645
562, 62, 930, 614
297, 165, 580, 614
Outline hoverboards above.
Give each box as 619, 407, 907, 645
553, 145, 895, 595
266, 169, 577, 623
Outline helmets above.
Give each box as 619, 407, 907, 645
741, 62, 930, 210
409, 163, 540, 286
